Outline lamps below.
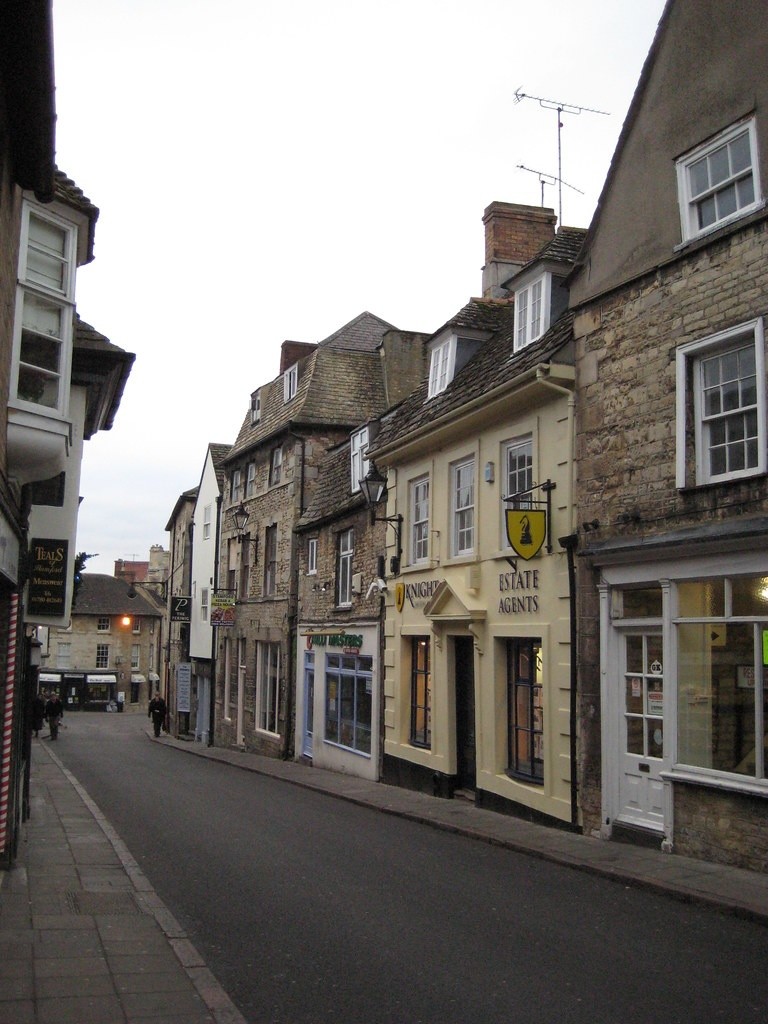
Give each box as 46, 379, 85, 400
126, 578, 168, 603
232, 499, 259, 566
358, 458, 404, 560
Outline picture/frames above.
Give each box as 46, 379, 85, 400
733, 663, 768, 690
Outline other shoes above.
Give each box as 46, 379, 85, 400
35, 733, 38, 736
155, 732, 160, 736
52, 736, 57, 740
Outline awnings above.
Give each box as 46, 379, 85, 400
131, 674, 146, 683
86, 674, 116, 683
149, 673, 159, 680
39, 674, 62, 683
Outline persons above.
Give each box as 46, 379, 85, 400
148, 691, 166, 737
32, 688, 64, 740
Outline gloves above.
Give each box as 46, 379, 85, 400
45, 718, 48, 722
61, 713, 63, 717
148, 712, 150, 718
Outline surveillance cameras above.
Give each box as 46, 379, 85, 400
377, 578, 387, 592
366, 582, 378, 601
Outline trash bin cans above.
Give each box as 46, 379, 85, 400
65, 695, 80, 711
116, 701, 123, 712
